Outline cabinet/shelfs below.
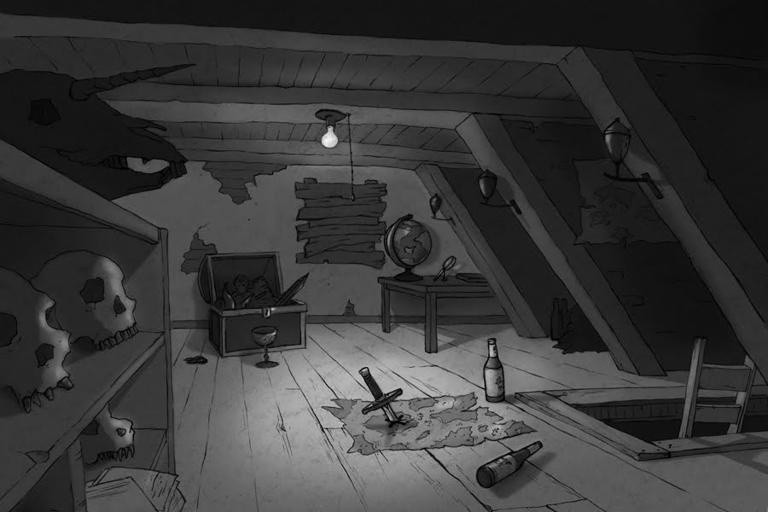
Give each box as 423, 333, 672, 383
0, 139, 177, 511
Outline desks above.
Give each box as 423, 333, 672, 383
376, 276, 496, 353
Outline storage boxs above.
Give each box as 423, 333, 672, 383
197, 251, 308, 358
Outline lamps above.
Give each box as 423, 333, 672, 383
314, 109, 347, 150
477, 169, 523, 214
601, 117, 664, 200
427, 193, 456, 225
